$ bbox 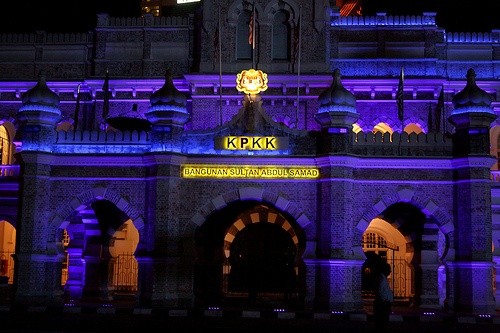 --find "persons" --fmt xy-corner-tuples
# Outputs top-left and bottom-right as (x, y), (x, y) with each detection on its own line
(242, 250), (394, 332)
